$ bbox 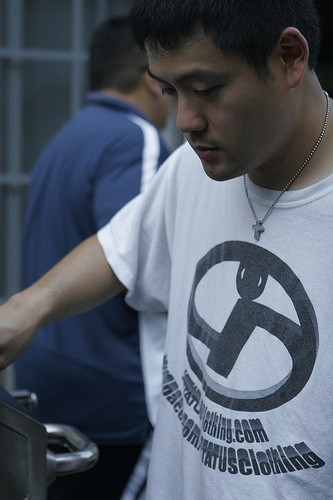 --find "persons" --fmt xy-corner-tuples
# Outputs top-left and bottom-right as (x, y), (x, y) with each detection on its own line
(0, 0), (333, 500)
(241, 90), (330, 241)
(13, 11), (168, 500)
